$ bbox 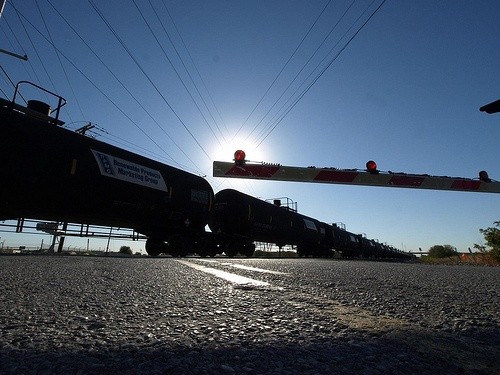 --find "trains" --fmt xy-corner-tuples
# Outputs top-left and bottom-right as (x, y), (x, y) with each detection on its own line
(0, 81), (423, 263)
